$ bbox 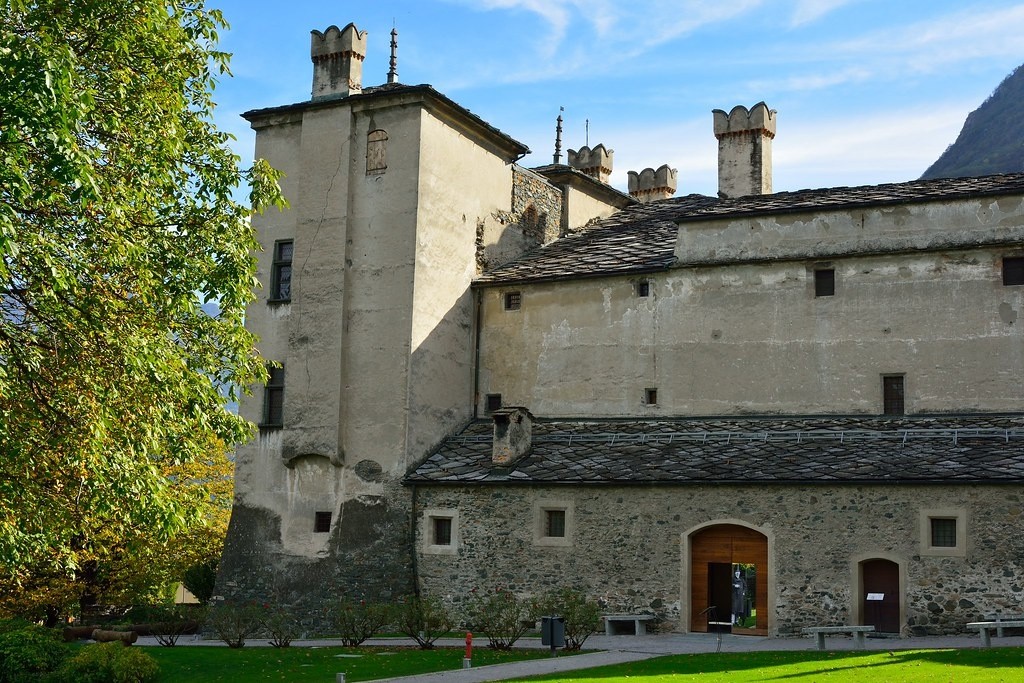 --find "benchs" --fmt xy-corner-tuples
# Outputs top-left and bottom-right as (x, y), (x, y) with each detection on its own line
(601, 615), (655, 636)
(966, 614), (1024, 646)
(802, 625), (875, 650)
